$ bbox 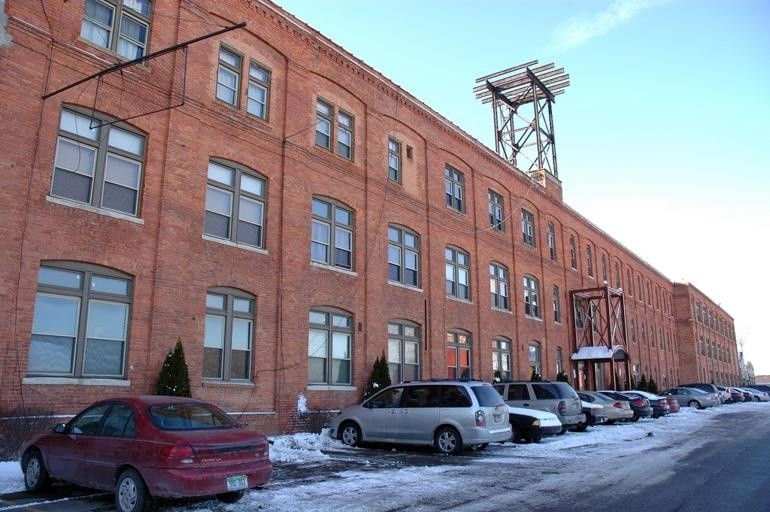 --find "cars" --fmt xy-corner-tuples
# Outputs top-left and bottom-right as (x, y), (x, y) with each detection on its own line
(493, 381), (770, 443)
(330, 379), (513, 456)
(18, 395), (273, 511)
